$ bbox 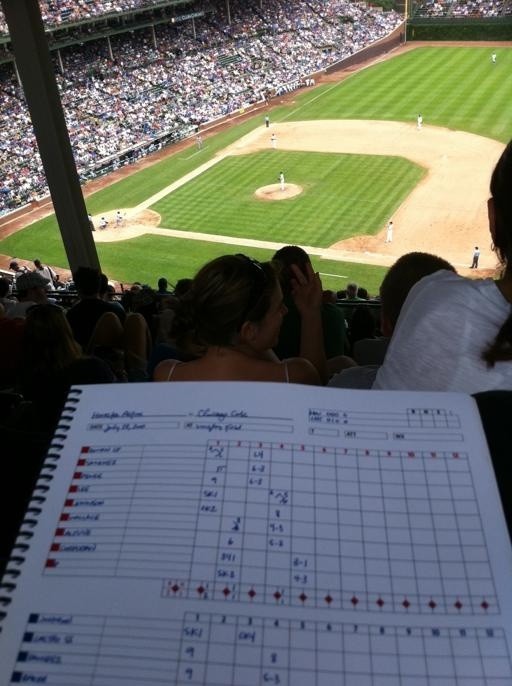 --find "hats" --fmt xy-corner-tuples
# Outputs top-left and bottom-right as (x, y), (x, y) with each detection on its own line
(16, 272), (51, 288)
